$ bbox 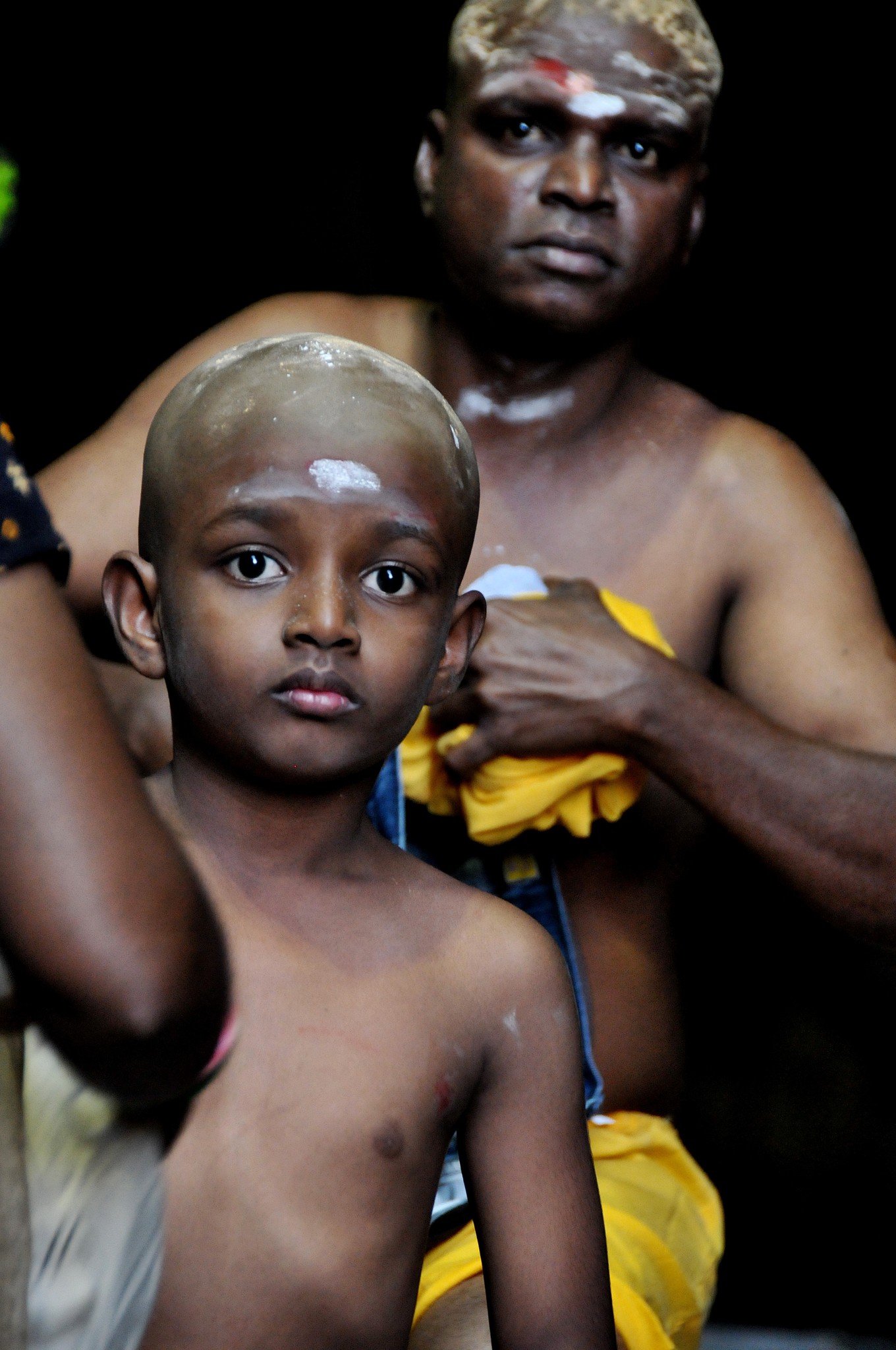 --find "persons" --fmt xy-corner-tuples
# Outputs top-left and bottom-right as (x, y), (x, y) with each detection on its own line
(35, 331), (633, 1348)
(0, 409), (240, 1343)
(0, 0), (896, 1348)
